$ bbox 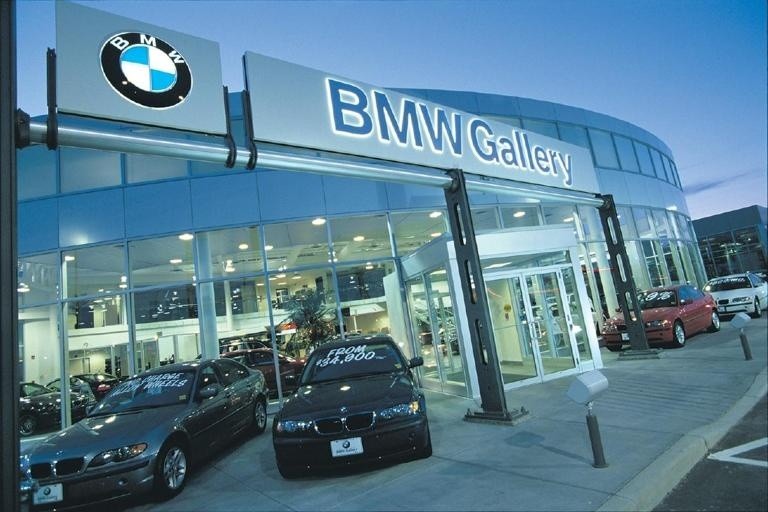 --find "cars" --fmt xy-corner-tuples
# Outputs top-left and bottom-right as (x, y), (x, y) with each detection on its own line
(599, 268), (767, 355)
(435, 316), (485, 359)
(521, 291), (612, 358)
(268, 333), (434, 486)
(19, 354), (272, 512)
(15, 333), (307, 442)
